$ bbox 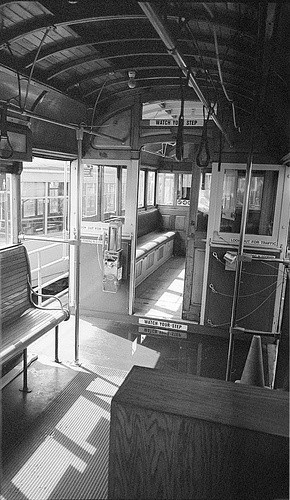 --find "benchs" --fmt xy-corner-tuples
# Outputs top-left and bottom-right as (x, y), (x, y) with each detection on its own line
(110, 206), (178, 269)
(0, 243), (70, 398)
